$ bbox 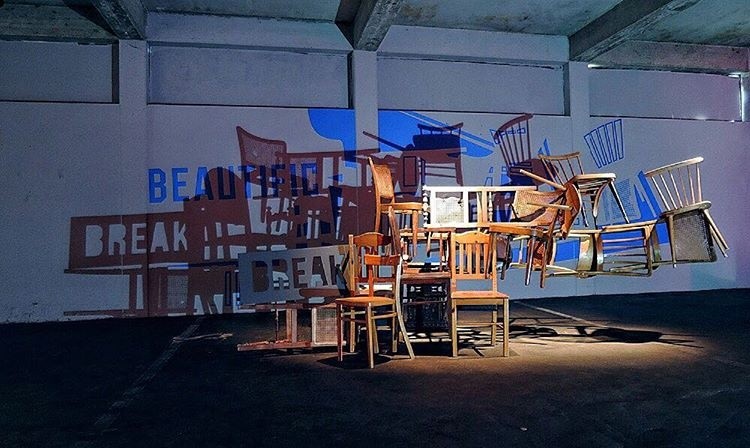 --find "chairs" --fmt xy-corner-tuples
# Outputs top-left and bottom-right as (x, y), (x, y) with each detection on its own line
(60, 113), (730, 369)
